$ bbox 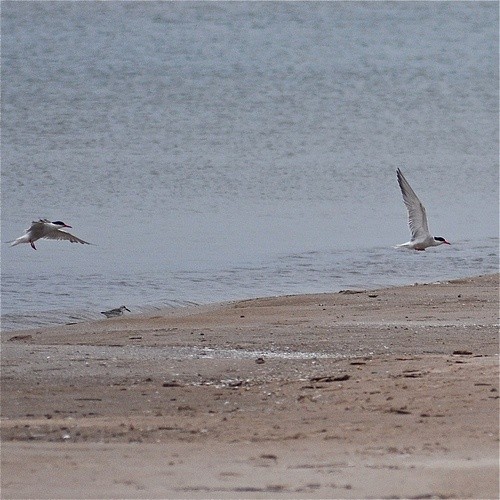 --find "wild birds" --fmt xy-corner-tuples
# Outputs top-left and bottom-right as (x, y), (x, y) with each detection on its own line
(100, 305), (131, 319)
(5, 217), (95, 250)
(392, 167), (451, 251)
